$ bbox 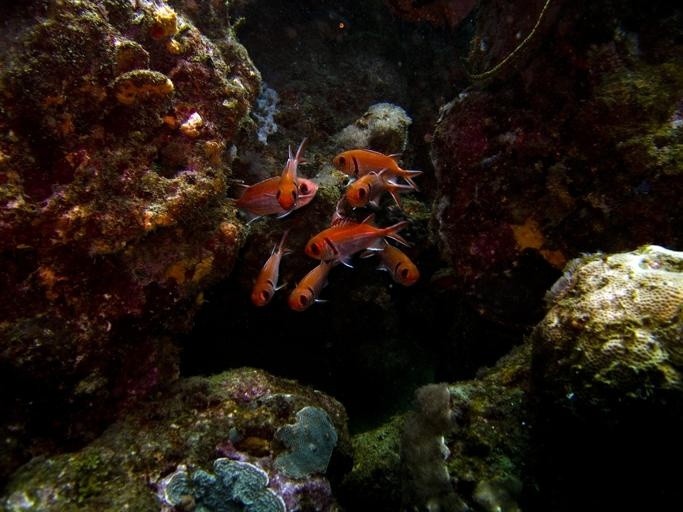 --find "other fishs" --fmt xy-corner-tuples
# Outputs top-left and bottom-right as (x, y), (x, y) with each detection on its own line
(196, 137), (424, 313)
